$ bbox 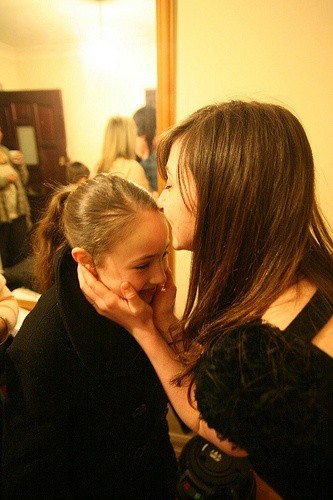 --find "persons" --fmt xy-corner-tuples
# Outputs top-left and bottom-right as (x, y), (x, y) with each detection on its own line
(0, 105), (159, 356)
(77, 102), (333, 500)
(0, 174), (177, 500)
